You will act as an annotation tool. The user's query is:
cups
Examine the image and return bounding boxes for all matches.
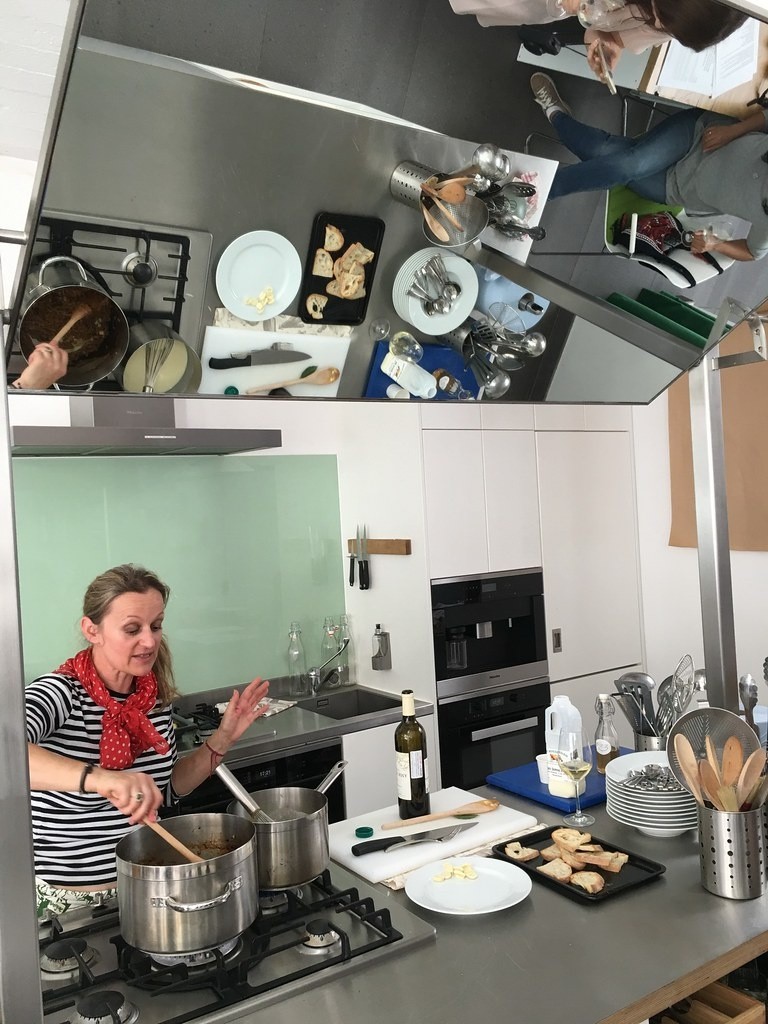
[536,755,550,784]
[386,384,410,399]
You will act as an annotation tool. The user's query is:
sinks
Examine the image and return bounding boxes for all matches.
[289,686,402,720]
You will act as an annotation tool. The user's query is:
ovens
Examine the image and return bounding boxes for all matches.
[158,736,347,826]
[431,567,552,792]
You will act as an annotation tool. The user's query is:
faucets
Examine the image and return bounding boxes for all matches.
[307,666,343,696]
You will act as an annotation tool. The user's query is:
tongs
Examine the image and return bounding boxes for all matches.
[740,657,768,747]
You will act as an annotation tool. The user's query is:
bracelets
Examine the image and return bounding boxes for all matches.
[80,761,97,794]
[204,740,224,778]
[11,382,22,389]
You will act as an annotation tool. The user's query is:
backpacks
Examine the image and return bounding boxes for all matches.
[613,211,724,288]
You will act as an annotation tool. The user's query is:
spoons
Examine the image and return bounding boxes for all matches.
[432,256,461,297]
[420,268,437,315]
[426,262,457,300]
[382,800,498,830]
[409,281,453,315]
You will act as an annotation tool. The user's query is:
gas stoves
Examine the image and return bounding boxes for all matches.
[5,218,191,394]
[171,703,276,754]
[36,861,435,1024]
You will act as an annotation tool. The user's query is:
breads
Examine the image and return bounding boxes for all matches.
[305,225,375,320]
[505,827,629,894]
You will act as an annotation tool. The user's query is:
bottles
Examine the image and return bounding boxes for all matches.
[545,694,620,798]
[394,691,431,820]
[321,615,357,689]
[288,623,308,696]
[372,624,386,658]
[379,352,470,400]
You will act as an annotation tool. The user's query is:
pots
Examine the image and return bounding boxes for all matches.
[14,254,203,396]
[116,788,327,954]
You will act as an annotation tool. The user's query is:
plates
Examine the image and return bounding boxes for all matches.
[392,247,480,337]
[405,856,533,915]
[215,211,387,326]
[605,751,698,837]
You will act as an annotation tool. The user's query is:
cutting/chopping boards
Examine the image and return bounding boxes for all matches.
[198,325,352,398]
[328,786,537,885]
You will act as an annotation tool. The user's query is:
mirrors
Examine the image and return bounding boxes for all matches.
[6,0,768,403]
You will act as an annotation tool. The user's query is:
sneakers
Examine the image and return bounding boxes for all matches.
[530,73,570,123]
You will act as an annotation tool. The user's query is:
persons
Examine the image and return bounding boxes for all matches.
[6,342,69,389]
[449,0,750,82]
[25,562,271,923]
[530,72,768,261]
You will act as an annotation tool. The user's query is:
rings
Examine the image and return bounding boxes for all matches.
[45,347,53,352]
[134,792,144,801]
[709,131,711,134]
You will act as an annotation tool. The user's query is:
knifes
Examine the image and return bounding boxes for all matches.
[209,350,313,370]
[351,822,478,857]
[349,526,370,590]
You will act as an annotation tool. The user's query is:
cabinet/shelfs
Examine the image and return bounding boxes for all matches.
[334,406,647,815]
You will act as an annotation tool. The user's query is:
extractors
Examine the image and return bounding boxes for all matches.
[9,396,284,458]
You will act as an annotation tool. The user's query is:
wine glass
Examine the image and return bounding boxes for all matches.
[681,221,734,247]
[546,0,625,30]
[558,727,596,827]
[369,317,423,364]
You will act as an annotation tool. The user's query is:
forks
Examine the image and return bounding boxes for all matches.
[231,342,292,360]
[385,827,462,852]
